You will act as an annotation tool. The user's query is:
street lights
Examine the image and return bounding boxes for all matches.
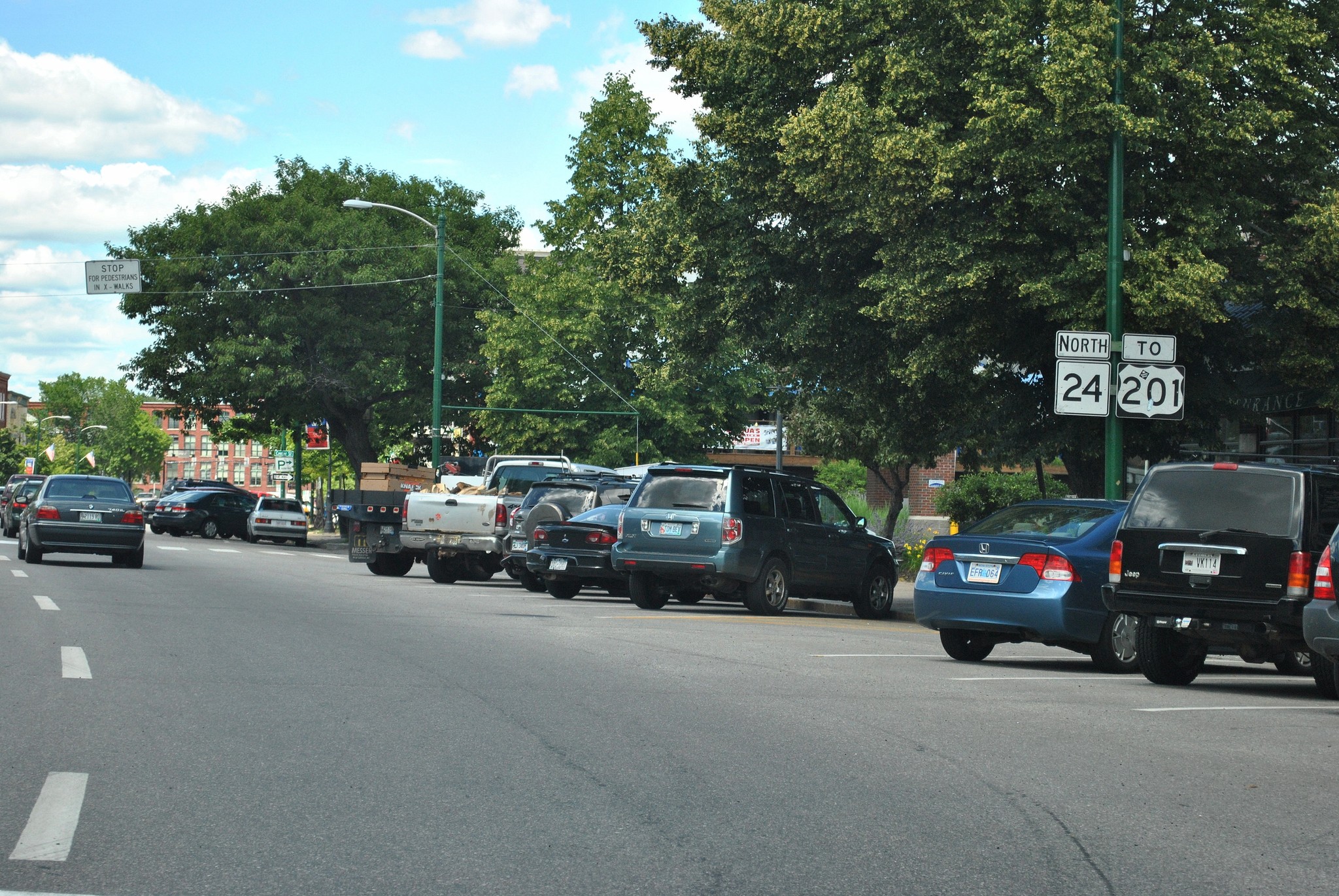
[341,199,449,472]
[34,411,72,473]
[75,424,108,474]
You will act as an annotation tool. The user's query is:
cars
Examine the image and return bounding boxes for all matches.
[134,476,277,541]
[0,474,47,537]
[911,498,1140,673]
[12,474,145,568]
[1300,521,1338,695]
[612,462,902,616]
[246,497,308,546]
[530,502,635,600]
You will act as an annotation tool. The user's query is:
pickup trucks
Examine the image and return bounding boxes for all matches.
[401,453,623,589]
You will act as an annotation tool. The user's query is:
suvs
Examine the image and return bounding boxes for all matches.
[1102,462,1339,687]
[503,478,639,590]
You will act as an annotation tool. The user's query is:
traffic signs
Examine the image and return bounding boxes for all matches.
[273,473,294,482]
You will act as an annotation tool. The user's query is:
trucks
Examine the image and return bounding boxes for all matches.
[327,451,497,586]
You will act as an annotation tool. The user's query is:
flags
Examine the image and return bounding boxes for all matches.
[46,444,55,461]
[86,450,96,468]
